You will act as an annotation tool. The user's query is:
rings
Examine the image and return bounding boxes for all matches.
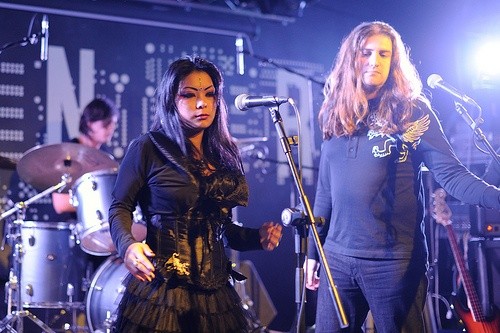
[276,242,279,246]
[134,260,138,265]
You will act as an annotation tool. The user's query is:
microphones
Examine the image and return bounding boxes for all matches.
[427,74,479,106]
[234,94,291,110]
[40,14,49,61]
[235,34,245,76]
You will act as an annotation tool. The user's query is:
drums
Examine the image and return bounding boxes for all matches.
[84,253,131,333]
[7,219,101,311]
[69,168,149,257]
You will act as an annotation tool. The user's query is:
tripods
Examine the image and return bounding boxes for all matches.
[0,178,71,332]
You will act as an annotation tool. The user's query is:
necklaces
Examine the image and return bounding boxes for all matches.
[194,157,208,168]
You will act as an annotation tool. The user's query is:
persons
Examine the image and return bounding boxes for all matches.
[107,56,284,333]
[303,20,500,333]
[52,95,115,216]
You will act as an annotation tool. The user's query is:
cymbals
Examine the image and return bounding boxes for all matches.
[17,142,121,191]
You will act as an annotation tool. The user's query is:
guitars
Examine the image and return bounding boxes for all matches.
[431,188,500,333]
